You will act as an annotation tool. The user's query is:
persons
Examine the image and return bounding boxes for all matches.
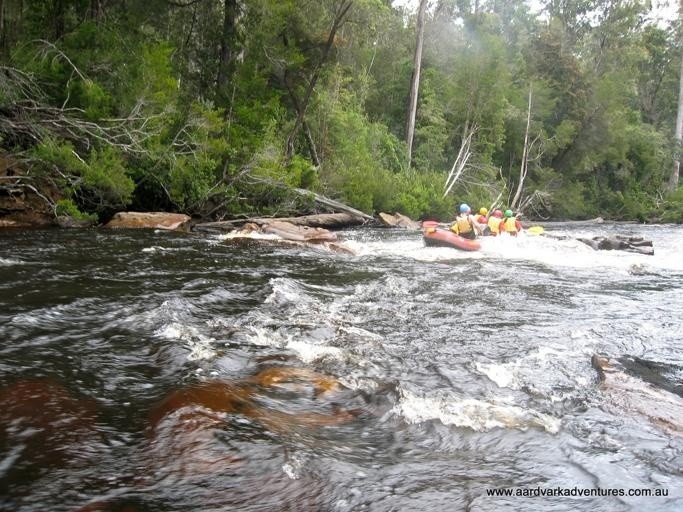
[449,203,522,240]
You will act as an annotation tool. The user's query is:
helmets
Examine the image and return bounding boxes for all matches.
[493,210,503,217]
[480,207,487,215]
[505,208,513,215]
[460,204,470,213]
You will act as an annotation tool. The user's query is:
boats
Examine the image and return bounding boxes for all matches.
[423,226,482,252]
